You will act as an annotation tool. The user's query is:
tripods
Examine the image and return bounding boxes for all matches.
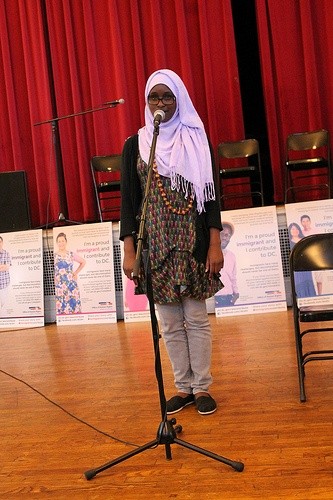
[33,104,117,229]
[83,124,244,481]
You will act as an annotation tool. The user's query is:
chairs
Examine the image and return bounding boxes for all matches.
[89,154,121,222]
[217,138,264,211]
[284,129,333,204]
[289,233,333,402]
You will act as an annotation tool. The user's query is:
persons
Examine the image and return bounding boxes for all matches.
[208,222,239,307]
[54,232,86,316]
[286,215,323,300]
[119,69,226,415]
[0,236,12,310]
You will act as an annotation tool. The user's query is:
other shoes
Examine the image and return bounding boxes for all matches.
[193,393,218,415]
[165,393,194,415]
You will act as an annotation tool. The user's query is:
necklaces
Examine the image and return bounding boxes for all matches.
[152,155,195,214]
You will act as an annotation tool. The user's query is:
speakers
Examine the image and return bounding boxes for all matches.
[0,170,32,234]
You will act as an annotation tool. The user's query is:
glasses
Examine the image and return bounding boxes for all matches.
[147,95,176,105]
[219,231,232,240]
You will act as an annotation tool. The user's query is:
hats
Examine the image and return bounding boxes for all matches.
[220,221,235,236]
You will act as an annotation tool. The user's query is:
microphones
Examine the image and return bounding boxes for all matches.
[103,99,125,105]
[153,110,166,125]
